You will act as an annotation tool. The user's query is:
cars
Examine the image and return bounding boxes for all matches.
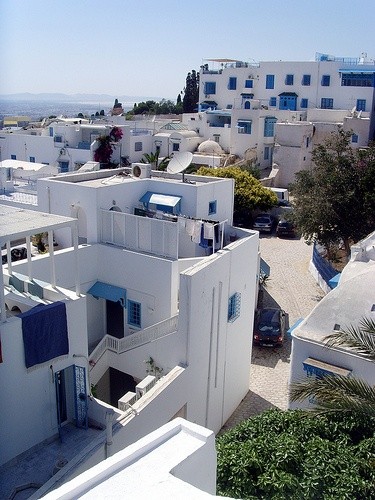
[232,210,251,228]
[276,218,297,238]
[253,213,275,232]
[253,308,285,347]
[278,198,294,209]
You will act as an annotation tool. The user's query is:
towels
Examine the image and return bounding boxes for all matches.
[154,210,222,249]
